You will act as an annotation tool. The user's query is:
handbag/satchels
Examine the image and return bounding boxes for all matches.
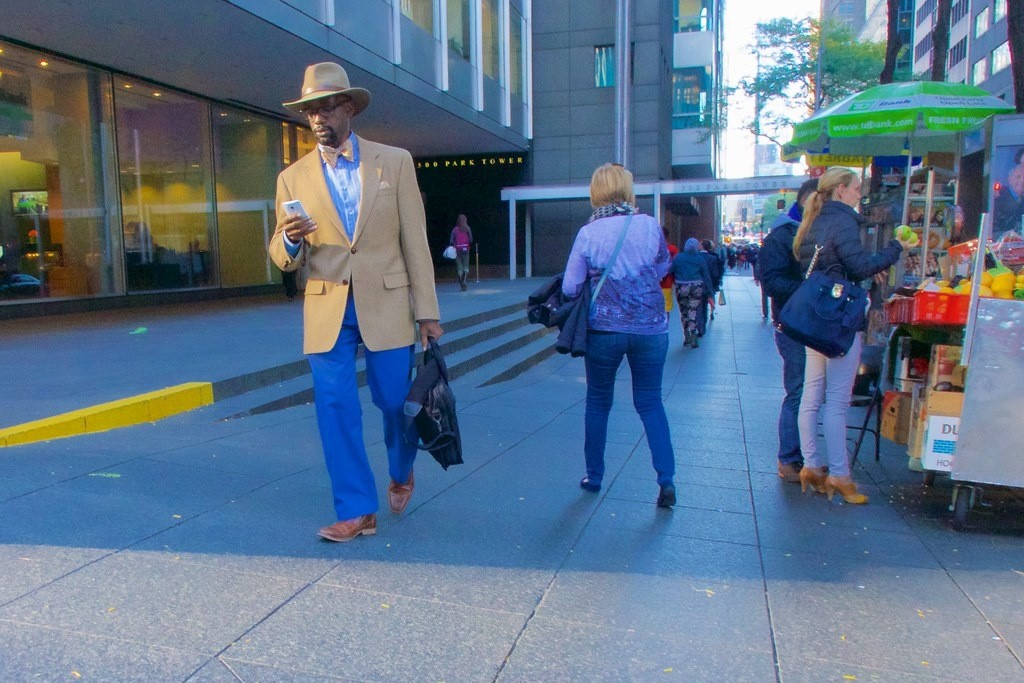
[442,242,457,260]
[775,263,869,359]
[403,337,464,471]
[718,289,726,306]
[705,301,712,332]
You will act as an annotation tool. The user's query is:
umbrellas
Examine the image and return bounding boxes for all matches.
[780,139,871,216]
[791,81,1014,286]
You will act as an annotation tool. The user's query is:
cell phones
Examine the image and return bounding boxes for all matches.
[281,200,309,219]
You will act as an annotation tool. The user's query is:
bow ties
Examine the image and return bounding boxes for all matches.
[321,138,355,168]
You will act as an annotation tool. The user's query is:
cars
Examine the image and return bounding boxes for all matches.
[0,274,50,297]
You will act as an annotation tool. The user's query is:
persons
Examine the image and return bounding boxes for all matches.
[450,214,473,292]
[792,168,920,504]
[661,225,777,348]
[562,164,677,507]
[268,61,443,543]
[757,178,830,485]
[906,209,945,277]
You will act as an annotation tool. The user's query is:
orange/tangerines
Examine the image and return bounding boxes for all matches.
[932,272,1024,300]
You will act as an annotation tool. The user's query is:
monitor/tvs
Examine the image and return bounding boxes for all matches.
[9,188,50,217]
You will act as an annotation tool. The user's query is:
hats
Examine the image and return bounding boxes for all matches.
[282,62,373,122]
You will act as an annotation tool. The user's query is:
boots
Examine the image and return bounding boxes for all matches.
[458,271,467,291]
[690,335,699,347]
[683,331,691,345]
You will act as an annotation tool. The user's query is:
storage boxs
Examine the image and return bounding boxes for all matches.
[948,238,994,262]
[881,336,966,472]
[884,291,971,326]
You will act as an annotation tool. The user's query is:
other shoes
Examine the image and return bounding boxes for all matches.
[777,459,800,482]
[657,481,677,508]
[580,476,601,492]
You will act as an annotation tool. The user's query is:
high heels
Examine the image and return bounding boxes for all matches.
[799,466,837,494]
[824,477,869,504]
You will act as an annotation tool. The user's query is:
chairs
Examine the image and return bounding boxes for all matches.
[815,345,886,471]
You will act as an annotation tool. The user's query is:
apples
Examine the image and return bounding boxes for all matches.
[894,225,918,245]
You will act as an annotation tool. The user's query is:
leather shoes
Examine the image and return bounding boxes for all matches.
[316,513,377,542]
[387,469,413,513]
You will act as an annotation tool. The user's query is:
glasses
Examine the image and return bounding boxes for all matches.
[299,99,352,121]
